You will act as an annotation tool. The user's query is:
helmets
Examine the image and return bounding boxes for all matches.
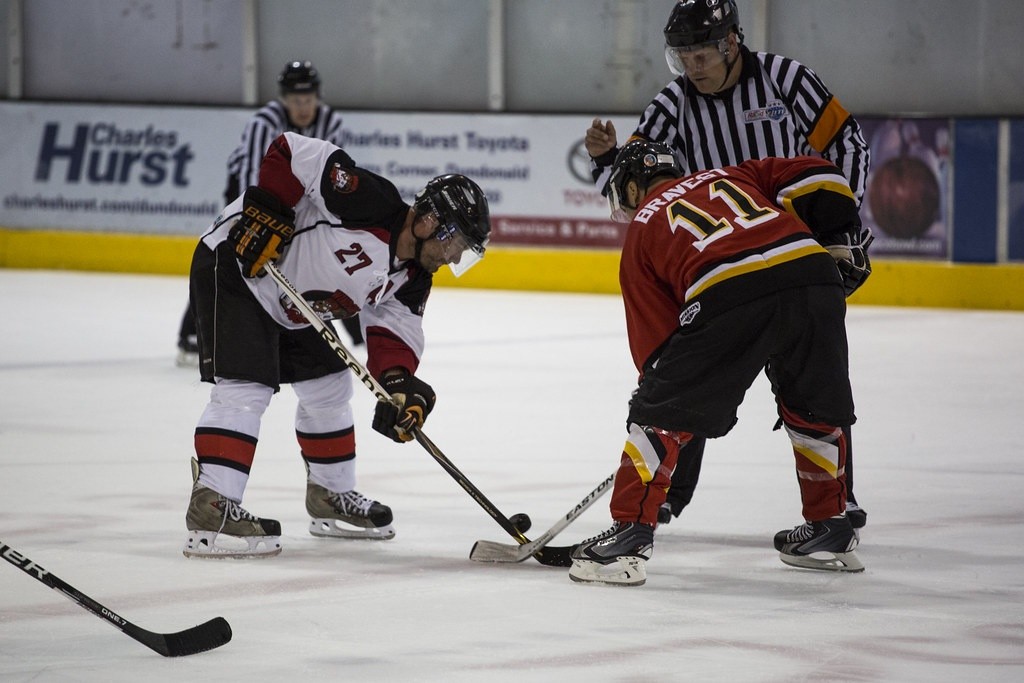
[663,0,739,47]
[601,139,685,203]
[276,61,321,95]
[415,173,491,258]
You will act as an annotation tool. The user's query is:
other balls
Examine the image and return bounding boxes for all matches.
[509,513,532,534]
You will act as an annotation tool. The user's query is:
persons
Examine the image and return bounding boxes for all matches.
[178,59,345,354]
[569,140,871,566]
[186,131,491,537]
[585,0,871,528]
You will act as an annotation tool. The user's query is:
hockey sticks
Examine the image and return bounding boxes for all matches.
[468,248,851,564]
[1,539,235,659]
[239,218,575,568]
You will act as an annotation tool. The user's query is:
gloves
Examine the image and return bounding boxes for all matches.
[227,186,296,279]
[816,226,876,297]
[372,374,436,443]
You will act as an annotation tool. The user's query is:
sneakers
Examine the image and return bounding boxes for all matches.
[774,511,865,574]
[183,456,282,559]
[301,450,396,540]
[569,520,655,587]
